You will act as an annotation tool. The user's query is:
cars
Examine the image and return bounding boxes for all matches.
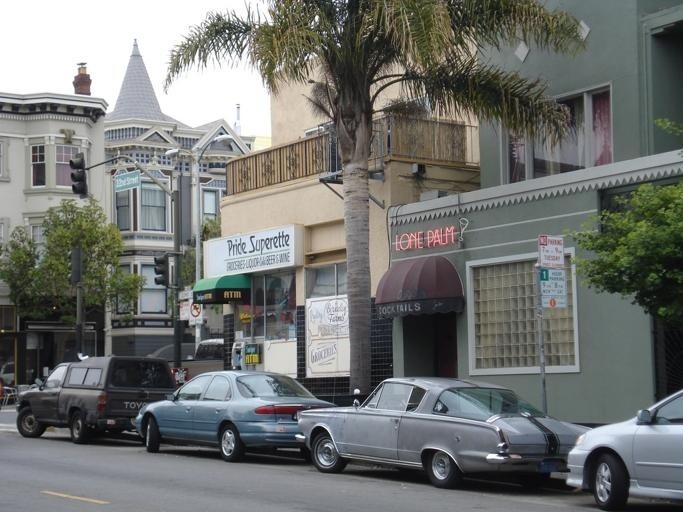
[564,388,682,511]
[1,361,15,386]
[134,370,340,462]
[295,374,593,486]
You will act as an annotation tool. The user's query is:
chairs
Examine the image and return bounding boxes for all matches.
[2,386,18,406]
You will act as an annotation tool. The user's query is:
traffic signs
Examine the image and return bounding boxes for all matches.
[114,169,141,192]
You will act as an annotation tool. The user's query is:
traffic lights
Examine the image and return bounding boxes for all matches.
[153,254,169,287]
[68,247,82,283]
[70,154,88,196]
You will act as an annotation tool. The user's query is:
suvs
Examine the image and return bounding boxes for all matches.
[15,355,180,446]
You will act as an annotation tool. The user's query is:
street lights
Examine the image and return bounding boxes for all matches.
[164,133,235,360]
